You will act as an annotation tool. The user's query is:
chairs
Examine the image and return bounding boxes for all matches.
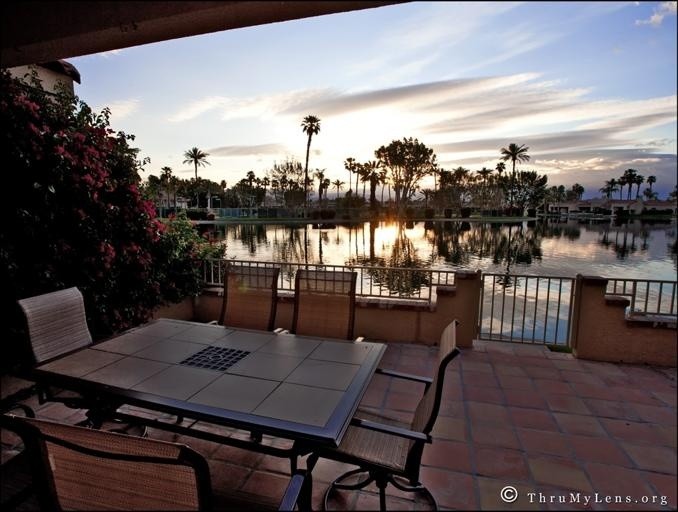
[0,259,461,511]
